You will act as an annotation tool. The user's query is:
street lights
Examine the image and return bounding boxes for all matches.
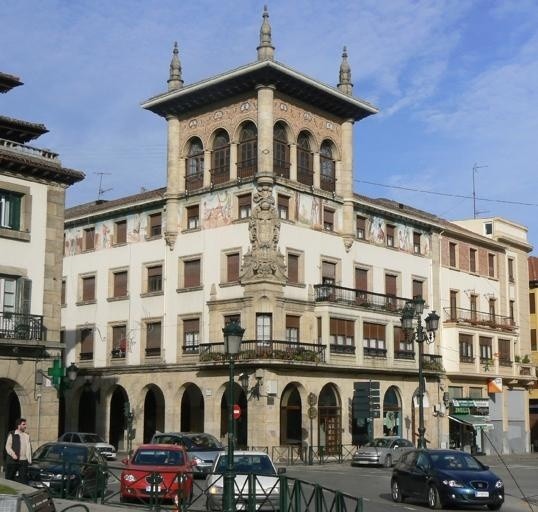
[221,319,245,511]
[401,294,441,452]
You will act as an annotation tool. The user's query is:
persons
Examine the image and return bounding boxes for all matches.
[5,418,32,484]
[448,457,463,468]
[376,441,384,447]
[237,458,252,472]
[164,453,181,464]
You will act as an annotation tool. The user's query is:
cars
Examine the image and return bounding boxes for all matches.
[207,451,288,511]
[148,430,224,478]
[17,442,108,501]
[352,436,414,469]
[120,444,197,505]
[59,431,116,460]
[392,449,506,512]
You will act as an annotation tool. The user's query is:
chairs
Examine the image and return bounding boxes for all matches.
[16,487,89,512]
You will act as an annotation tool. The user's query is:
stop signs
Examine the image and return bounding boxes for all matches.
[233,405,241,419]
